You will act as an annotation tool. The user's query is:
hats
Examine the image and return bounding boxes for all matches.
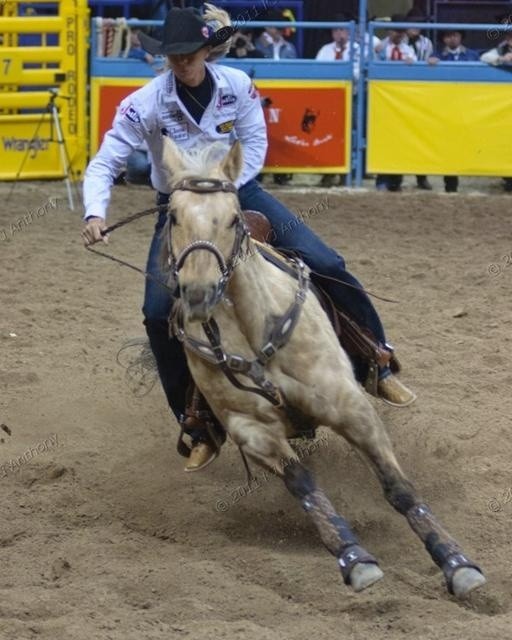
[254,8,291,22]
[401,7,430,22]
[137,7,234,57]
[386,12,407,32]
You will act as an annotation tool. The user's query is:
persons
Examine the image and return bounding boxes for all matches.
[80,7,418,472]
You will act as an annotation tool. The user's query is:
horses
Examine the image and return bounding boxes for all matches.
[158,133,489,600]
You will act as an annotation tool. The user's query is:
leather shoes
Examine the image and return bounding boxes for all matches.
[183,439,216,473]
[417,178,430,190]
[362,374,416,408]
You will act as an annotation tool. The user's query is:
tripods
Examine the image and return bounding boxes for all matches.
[6,104,81,210]
